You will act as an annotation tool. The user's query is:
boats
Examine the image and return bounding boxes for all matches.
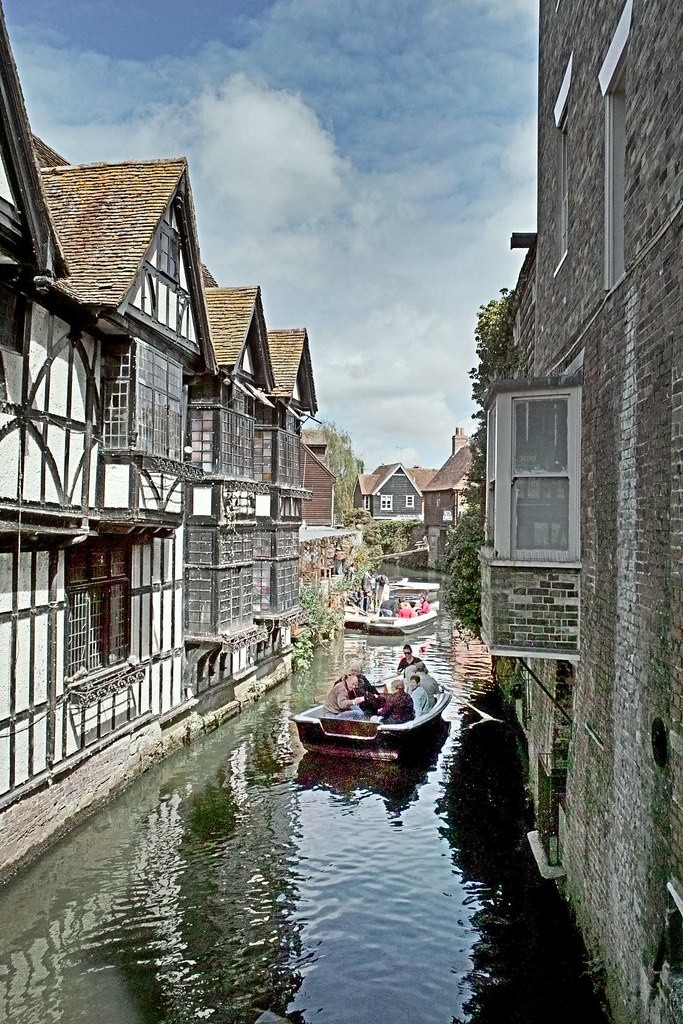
[343,608,439,636]
[290,675,452,761]
[390,581,440,594]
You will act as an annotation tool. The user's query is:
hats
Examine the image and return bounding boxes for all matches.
[416,662,426,669]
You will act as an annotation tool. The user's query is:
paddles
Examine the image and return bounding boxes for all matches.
[436,683,494,720]
[313,674,399,701]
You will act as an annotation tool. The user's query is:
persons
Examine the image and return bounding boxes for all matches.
[362,569,430,619]
[324,643,439,725]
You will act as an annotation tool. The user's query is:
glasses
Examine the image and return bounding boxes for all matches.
[404,652,411,654]
